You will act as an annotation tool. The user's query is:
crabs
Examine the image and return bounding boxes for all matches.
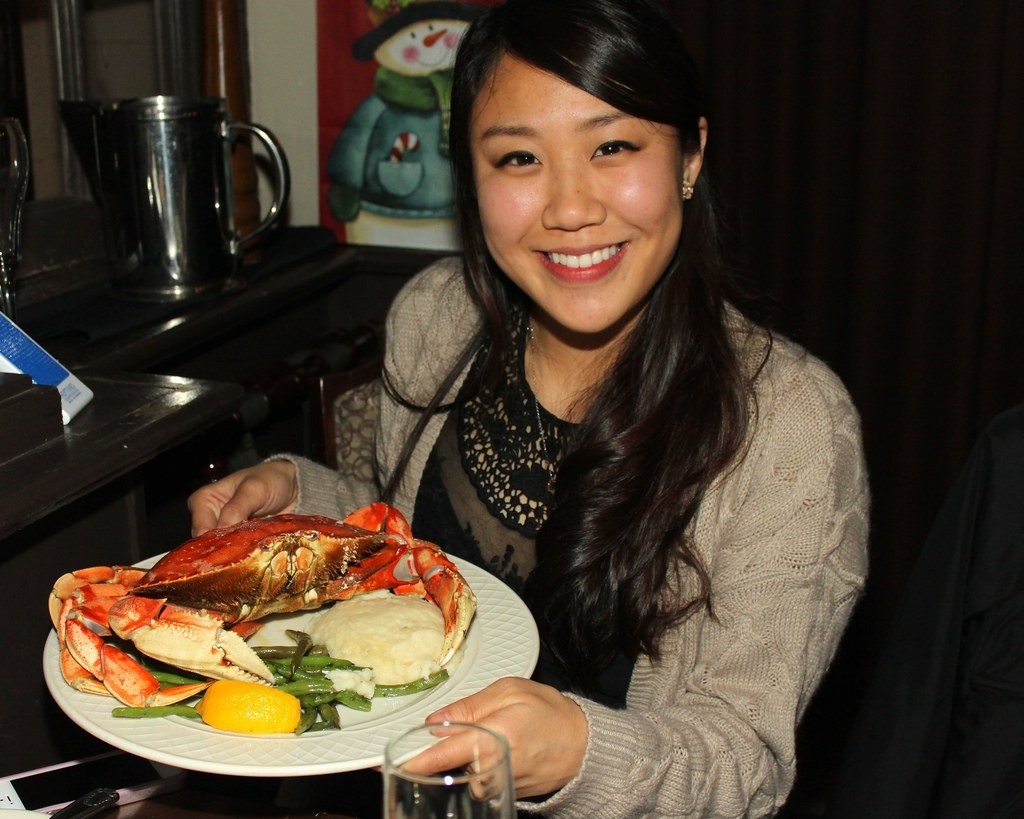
[48,502,477,707]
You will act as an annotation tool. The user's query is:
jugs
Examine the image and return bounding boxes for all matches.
[56,91,289,306]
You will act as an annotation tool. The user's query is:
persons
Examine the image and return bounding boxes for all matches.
[188,0,869,819]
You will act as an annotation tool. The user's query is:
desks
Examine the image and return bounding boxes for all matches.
[2,246,353,817]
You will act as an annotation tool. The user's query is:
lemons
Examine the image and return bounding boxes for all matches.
[194,679,301,736]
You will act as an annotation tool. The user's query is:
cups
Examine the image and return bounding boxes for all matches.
[382,720,518,819]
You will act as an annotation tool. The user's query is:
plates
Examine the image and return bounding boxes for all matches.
[41,550,540,779]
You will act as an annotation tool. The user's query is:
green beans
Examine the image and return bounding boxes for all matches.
[110,628,450,737]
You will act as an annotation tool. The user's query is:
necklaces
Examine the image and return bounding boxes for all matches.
[526,316,559,495]
